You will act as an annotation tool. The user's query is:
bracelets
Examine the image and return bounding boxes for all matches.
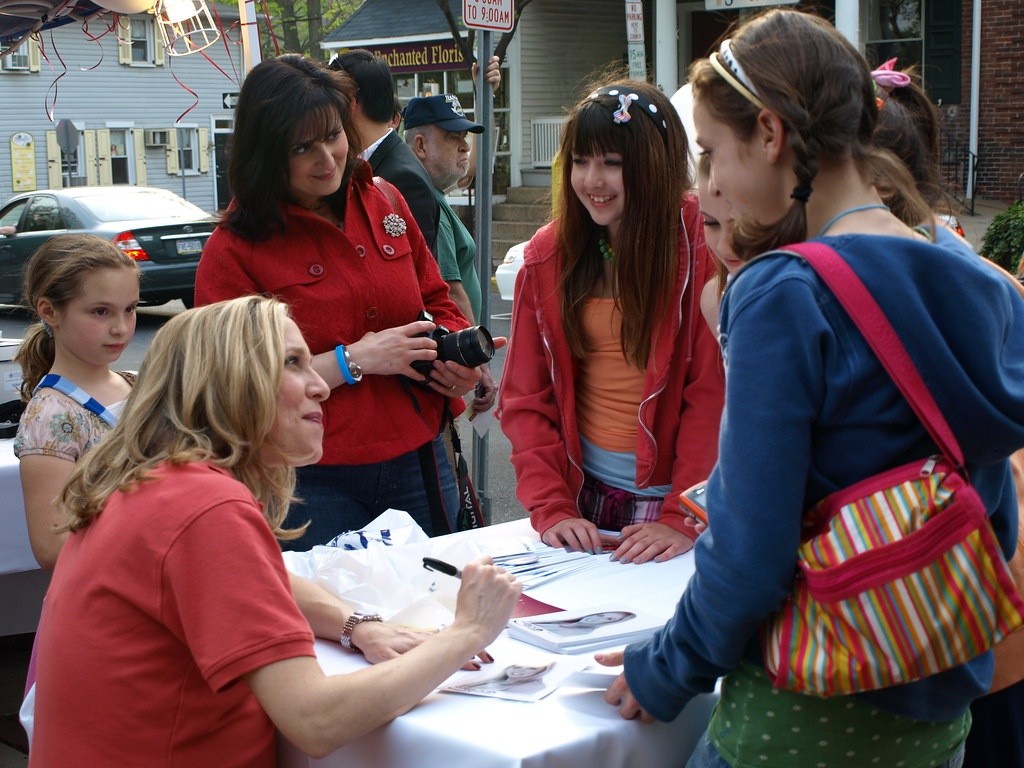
[335,345,358,385]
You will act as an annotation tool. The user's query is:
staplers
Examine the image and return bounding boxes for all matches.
[600,533,619,551]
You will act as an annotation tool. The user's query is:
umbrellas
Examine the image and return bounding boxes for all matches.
[670,82,703,194]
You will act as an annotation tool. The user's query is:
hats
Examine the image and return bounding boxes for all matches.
[403,94,485,134]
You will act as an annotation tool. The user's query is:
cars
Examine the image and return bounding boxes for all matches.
[0,185,221,309]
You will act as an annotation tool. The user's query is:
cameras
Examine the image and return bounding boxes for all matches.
[398,310,495,393]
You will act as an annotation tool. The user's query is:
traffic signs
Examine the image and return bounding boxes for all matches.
[222,92,241,110]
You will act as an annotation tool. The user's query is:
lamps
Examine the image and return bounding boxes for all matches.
[152,0,221,57]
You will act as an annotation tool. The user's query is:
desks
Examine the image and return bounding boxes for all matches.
[19,518,722,768]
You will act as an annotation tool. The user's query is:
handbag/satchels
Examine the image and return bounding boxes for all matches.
[761,241,1024,700]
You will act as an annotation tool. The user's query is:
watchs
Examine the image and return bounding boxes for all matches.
[343,349,362,382]
[341,609,384,651]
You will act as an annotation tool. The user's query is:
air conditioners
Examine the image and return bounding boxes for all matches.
[144,129,170,147]
[2,53,30,70]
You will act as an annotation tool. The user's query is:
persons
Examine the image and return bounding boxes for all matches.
[493,81,723,565]
[462,662,556,695]
[25,297,523,768]
[194,50,507,552]
[594,12,1024,768]
[11,234,140,567]
[530,611,637,638]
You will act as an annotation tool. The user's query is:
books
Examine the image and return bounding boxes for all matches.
[437,597,667,703]
[492,536,601,592]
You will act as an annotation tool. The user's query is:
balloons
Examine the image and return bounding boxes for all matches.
[90,0,156,14]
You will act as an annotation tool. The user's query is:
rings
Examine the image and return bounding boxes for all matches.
[450,385,456,391]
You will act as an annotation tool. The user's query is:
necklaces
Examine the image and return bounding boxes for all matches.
[815,205,890,239]
[301,201,326,211]
[599,238,617,265]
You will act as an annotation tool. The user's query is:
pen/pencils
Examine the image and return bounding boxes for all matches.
[423,558,461,578]
